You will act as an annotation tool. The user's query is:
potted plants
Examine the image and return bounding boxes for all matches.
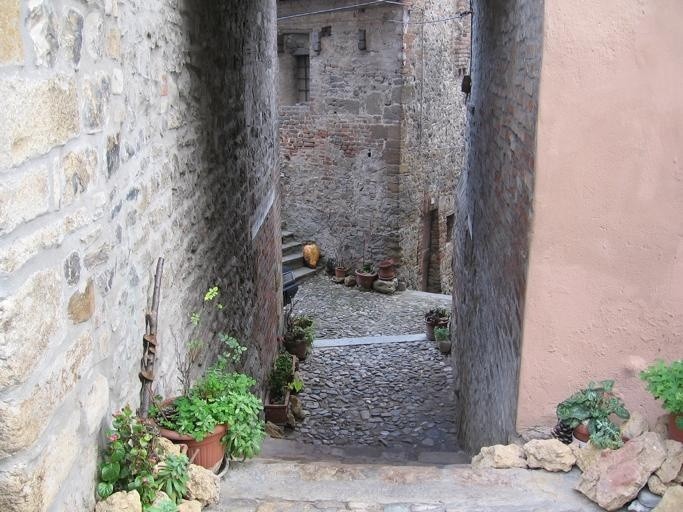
[637,354,682,446]
[258,348,300,431]
[129,271,269,487]
[279,290,315,361]
[327,257,396,293]
[422,305,451,342]
[553,378,631,453]
[430,323,452,353]
